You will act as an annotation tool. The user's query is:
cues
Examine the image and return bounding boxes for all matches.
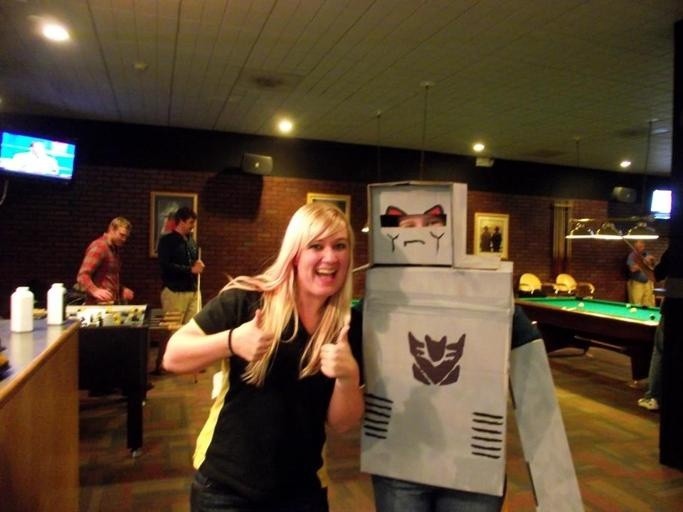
[192,247,202,383]
[574,335,626,352]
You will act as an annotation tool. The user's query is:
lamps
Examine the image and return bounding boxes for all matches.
[558,117,663,244]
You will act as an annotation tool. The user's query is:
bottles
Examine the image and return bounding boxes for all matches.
[10,287,32,332]
[46,283,67,325]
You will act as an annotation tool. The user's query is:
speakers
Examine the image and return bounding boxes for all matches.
[240,153,273,176]
[612,186,637,203]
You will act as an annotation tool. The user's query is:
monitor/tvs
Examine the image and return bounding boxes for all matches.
[0,127,78,186]
[646,184,672,223]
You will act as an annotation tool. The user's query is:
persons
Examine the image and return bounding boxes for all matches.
[161,200,365,508]
[347,215,541,511]
[491,225,502,252]
[480,224,491,252]
[156,206,207,374]
[637,233,673,410]
[14,142,59,176]
[76,216,156,392]
[626,240,655,308]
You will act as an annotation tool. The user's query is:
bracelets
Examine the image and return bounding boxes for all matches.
[229,328,234,357]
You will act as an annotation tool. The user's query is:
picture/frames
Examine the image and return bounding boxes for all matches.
[149,189,199,261]
[304,191,352,225]
[472,210,509,260]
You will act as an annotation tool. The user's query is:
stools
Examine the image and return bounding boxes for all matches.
[651,287,668,307]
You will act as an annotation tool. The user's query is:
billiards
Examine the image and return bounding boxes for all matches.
[649,313,655,320]
[631,307,637,312]
[626,304,632,308]
[578,302,584,308]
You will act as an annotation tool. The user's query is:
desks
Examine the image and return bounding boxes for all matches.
[0,316,86,512]
[509,293,665,387]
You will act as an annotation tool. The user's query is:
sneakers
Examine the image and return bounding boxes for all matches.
[638,398,660,410]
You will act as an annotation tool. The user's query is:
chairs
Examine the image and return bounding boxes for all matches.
[554,270,597,301]
[516,272,561,299]
